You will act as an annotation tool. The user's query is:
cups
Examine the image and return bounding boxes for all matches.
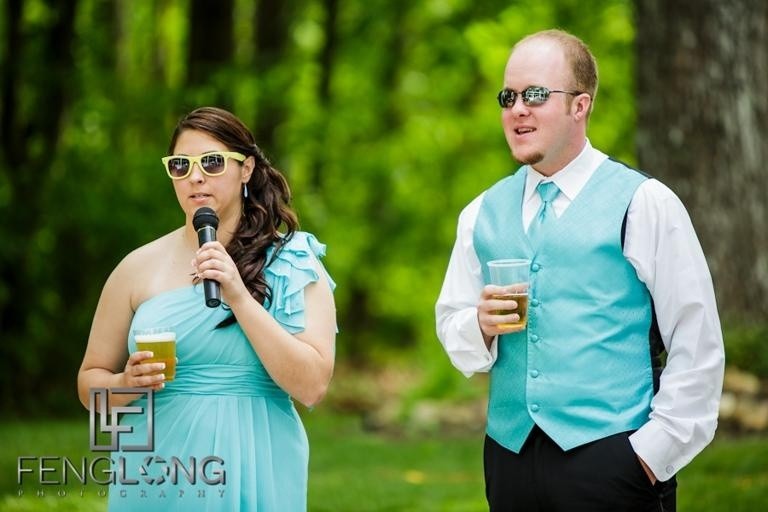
[486,258,532,329]
[131,326,177,383]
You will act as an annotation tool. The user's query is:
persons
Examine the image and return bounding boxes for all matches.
[76,105,340,512]
[435,30,727,512]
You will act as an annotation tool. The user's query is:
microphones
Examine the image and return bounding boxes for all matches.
[192,207,221,308]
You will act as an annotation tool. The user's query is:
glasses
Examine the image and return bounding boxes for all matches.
[495,85,593,110]
[161,149,248,181]
